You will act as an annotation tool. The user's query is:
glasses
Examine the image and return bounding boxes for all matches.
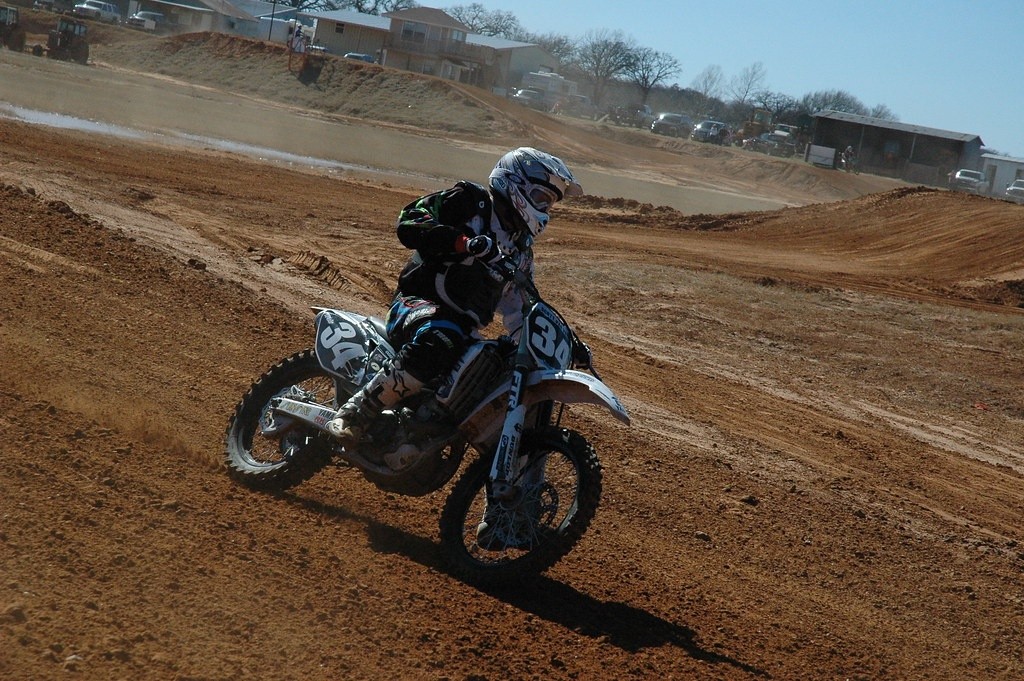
[526,184,558,214]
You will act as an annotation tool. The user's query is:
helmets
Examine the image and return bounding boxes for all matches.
[488,147,583,235]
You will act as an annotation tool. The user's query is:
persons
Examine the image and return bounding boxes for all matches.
[707,122,737,145]
[294,23,305,37]
[844,144,856,169]
[329,147,583,548]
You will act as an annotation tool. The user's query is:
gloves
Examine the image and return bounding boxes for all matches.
[465,235,502,269]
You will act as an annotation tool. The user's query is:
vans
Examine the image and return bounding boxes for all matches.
[774,124,795,138]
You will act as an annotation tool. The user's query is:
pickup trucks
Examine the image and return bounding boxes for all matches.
[613,103,657,128]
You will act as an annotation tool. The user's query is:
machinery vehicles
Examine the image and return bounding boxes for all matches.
[45,14,89,64]
[0,1,26,53]
[735,108,778,148]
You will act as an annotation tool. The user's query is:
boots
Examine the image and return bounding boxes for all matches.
[328,358,427,450]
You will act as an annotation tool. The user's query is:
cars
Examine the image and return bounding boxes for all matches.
[691,121,725,142]
[748,132,795,158]
[344,51,377,63]
[1005,179,1024,204]
[513,89,544,110]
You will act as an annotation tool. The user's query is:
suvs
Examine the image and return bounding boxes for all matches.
[951,169,990,195]
[128,11,165,32]
[72,0,121,25]
[650,115,693,138]
[562,94,600,121]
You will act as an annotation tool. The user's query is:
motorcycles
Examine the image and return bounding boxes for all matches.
[222,238,633,585]
[839,150,860,177]
